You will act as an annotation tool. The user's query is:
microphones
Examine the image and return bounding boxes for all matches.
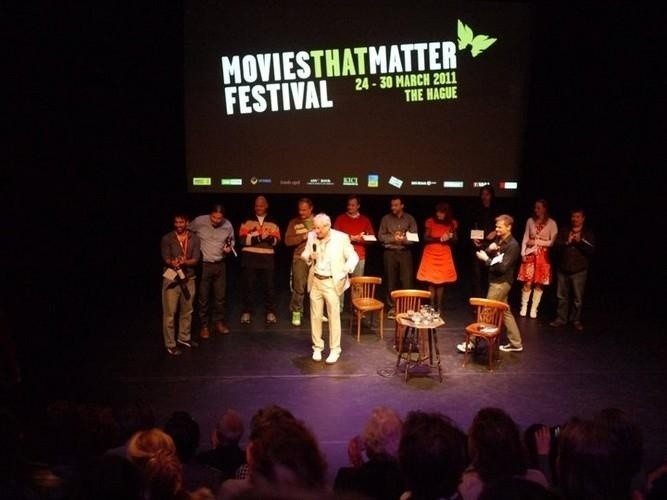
[313,244,316,265]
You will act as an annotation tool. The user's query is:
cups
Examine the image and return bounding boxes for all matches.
[406,305,441,326]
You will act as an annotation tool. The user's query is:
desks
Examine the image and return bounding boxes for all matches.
[396,313,445,383]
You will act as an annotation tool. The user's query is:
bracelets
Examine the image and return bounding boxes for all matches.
[188,260,193,266]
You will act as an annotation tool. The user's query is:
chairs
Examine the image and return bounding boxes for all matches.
[349,276,384,342]
[391,290,429,350]
[466,297,508,372]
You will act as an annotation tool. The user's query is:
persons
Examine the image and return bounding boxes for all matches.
[414,203,458,313]
[299,213,360,365]
[331,195,376,316]
[0,396,667,499]
[516,199,558,319]
[547,206,595,330]
[237,195,281,329]
[284,197,328,326]
[187,203,235,339]
[455,214,523,353]
[161,214,200,357]
[377,196,419,320]
[460,186,497,314]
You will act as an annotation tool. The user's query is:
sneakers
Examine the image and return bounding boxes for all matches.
[456,319,584,353]
[387,309,395,319]
[165,311,342,365]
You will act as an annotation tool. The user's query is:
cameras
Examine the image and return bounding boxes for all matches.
[551,425,561,438]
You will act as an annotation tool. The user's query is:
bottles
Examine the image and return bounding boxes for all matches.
[172,257,187,282]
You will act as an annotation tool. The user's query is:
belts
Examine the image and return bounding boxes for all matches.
[314,273,332,280]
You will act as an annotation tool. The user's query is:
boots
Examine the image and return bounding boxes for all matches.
[520,289,532,317]
[530,289,544,319]
